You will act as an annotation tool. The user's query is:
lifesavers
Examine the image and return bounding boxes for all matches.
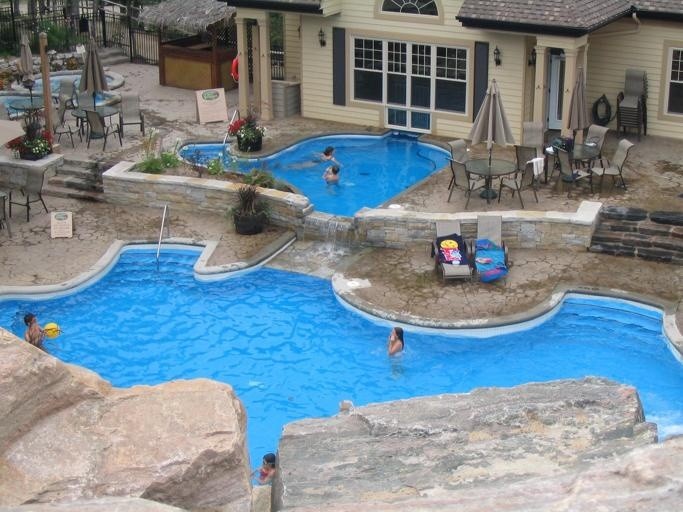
[441,240,458,248]
[232,58,239,80]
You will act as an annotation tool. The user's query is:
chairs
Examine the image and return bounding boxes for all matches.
[0,195,11,238]
[444,67,647,211]
[9,77,144,154]
[9,167,49,222]
[472,215,509,287]
[430,220,472,284]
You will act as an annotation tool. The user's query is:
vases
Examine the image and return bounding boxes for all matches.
[236,134,262,152]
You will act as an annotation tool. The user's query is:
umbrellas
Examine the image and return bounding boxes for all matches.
[78,39,109,111]
[566,63,590,150]
[21,35,36,102]
[467,78,516,166]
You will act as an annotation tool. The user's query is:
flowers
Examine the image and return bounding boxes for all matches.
[226,112,264,144]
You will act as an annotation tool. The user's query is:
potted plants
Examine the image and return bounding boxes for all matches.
[227,185,271,234]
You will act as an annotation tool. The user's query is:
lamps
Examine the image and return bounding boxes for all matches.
[316,26,327,48]
[493,44,501,67]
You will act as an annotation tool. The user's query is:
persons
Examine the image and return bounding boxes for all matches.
[289,146,344,170]
[323,166,340,185]
[24,313,47,352]
[250,453,276,485]
[388,327,404,355]
